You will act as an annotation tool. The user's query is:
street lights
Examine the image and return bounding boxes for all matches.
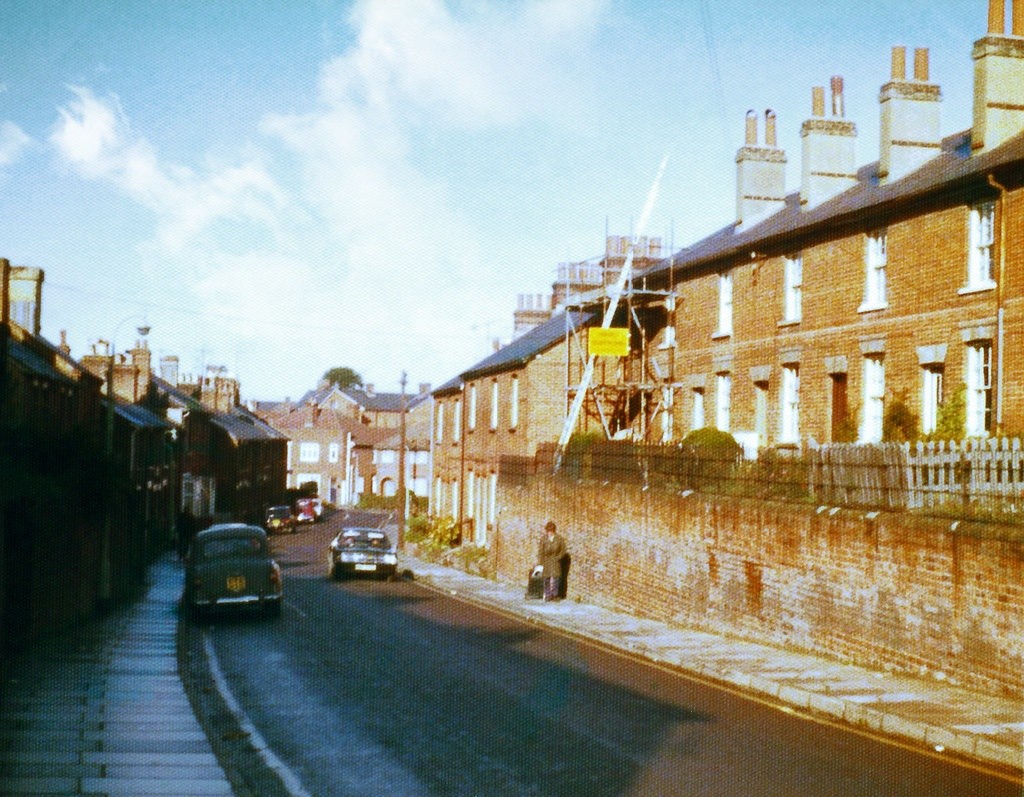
[343,431,355,519]
[101,315,150,599]
[396,445,410,520]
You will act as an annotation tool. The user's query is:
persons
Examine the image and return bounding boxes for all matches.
[174,506,197,562]
[538,522,567,601]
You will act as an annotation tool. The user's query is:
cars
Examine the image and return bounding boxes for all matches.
[184,523,284,618]
[265,506,297,534]
[328,527,399,580]
[298,498,323,522]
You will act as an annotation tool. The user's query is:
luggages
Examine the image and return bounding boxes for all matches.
[525,570,545,600]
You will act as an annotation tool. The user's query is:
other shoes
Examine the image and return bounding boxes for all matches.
[551,596,559,602]
[544,595,552,602]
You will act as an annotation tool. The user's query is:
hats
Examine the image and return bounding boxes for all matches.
[545,521,555,532]
